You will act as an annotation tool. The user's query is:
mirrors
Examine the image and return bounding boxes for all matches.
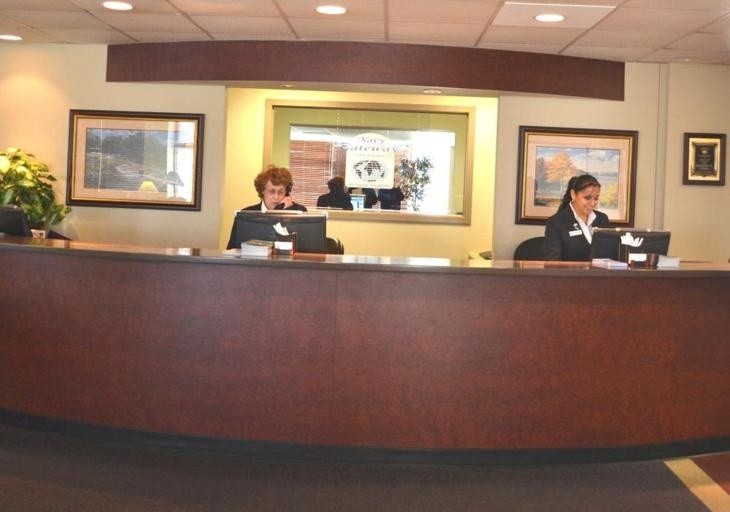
[261,98,476,224]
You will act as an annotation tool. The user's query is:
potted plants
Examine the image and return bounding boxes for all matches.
[0,147,70,238]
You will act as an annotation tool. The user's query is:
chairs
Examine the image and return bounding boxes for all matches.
[514,236,545,260]
[326,236,344,254]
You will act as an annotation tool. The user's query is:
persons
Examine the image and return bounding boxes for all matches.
[227,168,308,250]
[379,188,404,210]
[545,175,610,261]
[316,177,353,210]
[347,187,378,208]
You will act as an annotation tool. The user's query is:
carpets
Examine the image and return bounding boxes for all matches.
[664,452,730,512]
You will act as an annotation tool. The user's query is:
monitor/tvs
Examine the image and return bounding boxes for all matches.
[234,211,329,253]
[589,228,671,262]
[349,194,365,209]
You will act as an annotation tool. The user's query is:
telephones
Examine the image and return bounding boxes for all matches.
[274,183,293,210]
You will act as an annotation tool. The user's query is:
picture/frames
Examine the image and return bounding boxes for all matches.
[684,132,728,185]
[514,125,639,226]
[65,108,204,211]
